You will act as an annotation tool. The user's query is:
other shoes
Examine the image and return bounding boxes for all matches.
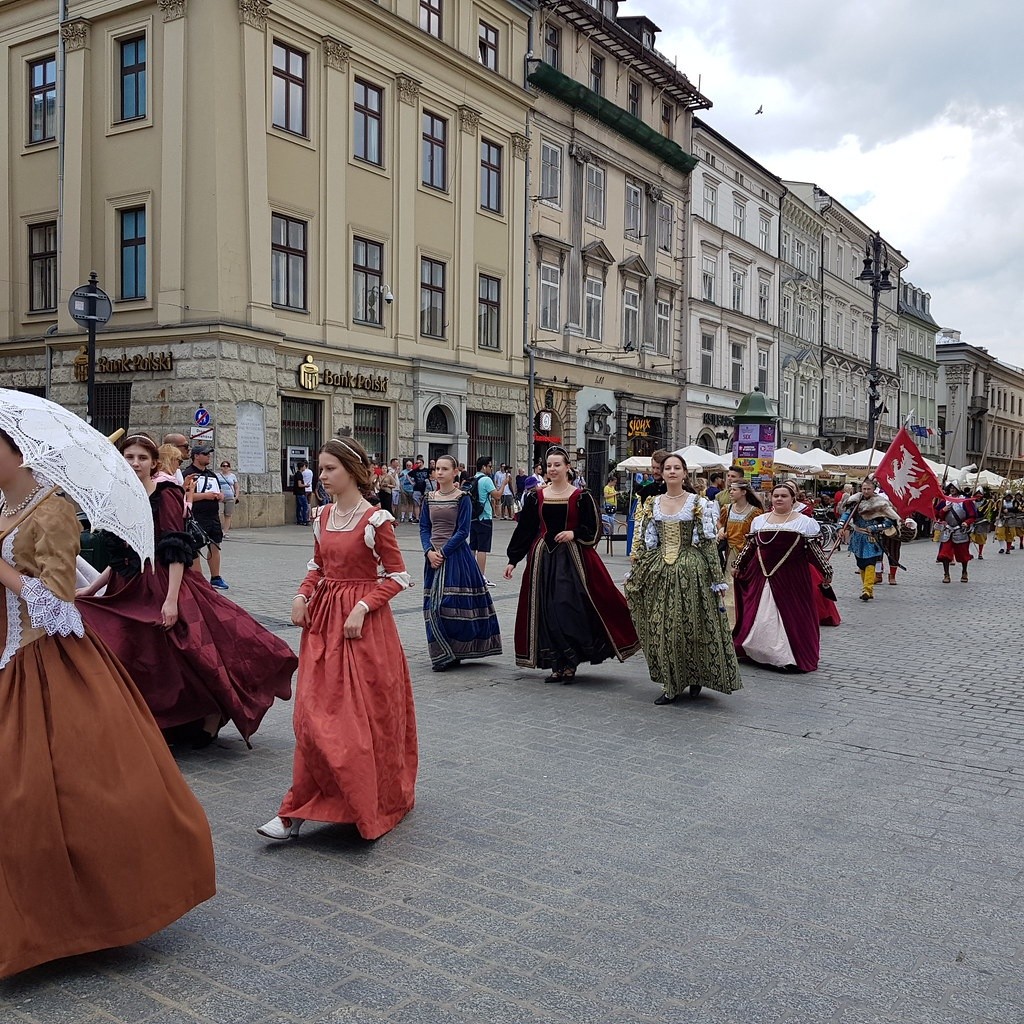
[545,667,562,682]
[654,691,677,705]
[297,521,311,526]
[400,519,404,523]
[689,681,700,697]
[508,517,514,521]
[500,517,509,520]
[192,717,222,750]
[412,519,419,524]
[562,663,576,682]
[483,578,496,587]
[859,542,1024,601]
[409,517,414,522]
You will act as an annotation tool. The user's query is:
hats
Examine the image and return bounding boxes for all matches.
[504,466,513,472]
[191,445,214,458]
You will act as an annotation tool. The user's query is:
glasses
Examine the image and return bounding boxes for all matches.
[487,465,492,469]
[200,452,208,456]
[222,465,230,468]
[176,443,189,450]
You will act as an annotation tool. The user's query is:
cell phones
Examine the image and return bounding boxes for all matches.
[193,475,198,483]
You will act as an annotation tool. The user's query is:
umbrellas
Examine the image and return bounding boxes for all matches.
[616,444,1024,487]
[0,388,155,575]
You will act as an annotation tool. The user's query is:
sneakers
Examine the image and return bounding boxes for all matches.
[210,578,228,589]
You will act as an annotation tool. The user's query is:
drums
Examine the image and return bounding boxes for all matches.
[884,523,917,543]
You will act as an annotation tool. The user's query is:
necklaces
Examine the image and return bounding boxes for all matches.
[734,502,749,514]
[550,484,570,494]
[759,509,794,544]
[438,487,456,495]
[330,497,363,530]
[2,484,44,516]
[665,490,685,499]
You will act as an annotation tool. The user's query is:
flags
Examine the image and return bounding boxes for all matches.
[877,430,945,522]
[910,425,933,438]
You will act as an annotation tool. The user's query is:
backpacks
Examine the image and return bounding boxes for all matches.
[461,476,488,519]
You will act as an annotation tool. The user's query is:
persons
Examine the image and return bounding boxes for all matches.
[294,461,331,526]
[0,429,216,978]
[623,454,745,704]
[371,450,772,631]
[153,433,239,589]
[785,478,1024,601]
[730,484,834,673]
[503,451,642,683]
[258,437,419,840]
[78,434,300,758]
[420,455,503,671]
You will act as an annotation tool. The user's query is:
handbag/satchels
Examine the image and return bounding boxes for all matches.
[186,507,210,549]
[606,506,616,514]
[234,495,240,504]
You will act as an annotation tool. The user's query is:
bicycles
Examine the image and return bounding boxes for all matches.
[817,520,841,552]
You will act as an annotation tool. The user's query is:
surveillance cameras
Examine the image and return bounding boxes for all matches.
[385,293,393,304]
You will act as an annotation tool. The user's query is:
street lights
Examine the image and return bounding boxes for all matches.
[855,230,897,449]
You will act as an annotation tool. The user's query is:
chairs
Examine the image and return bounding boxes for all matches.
[595,514,628,556]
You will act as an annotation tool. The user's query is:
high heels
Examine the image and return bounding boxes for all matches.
[338,820,358,847]
[257,817,305,840]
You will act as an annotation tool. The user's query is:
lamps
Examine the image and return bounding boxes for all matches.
[611,435,617,445]
